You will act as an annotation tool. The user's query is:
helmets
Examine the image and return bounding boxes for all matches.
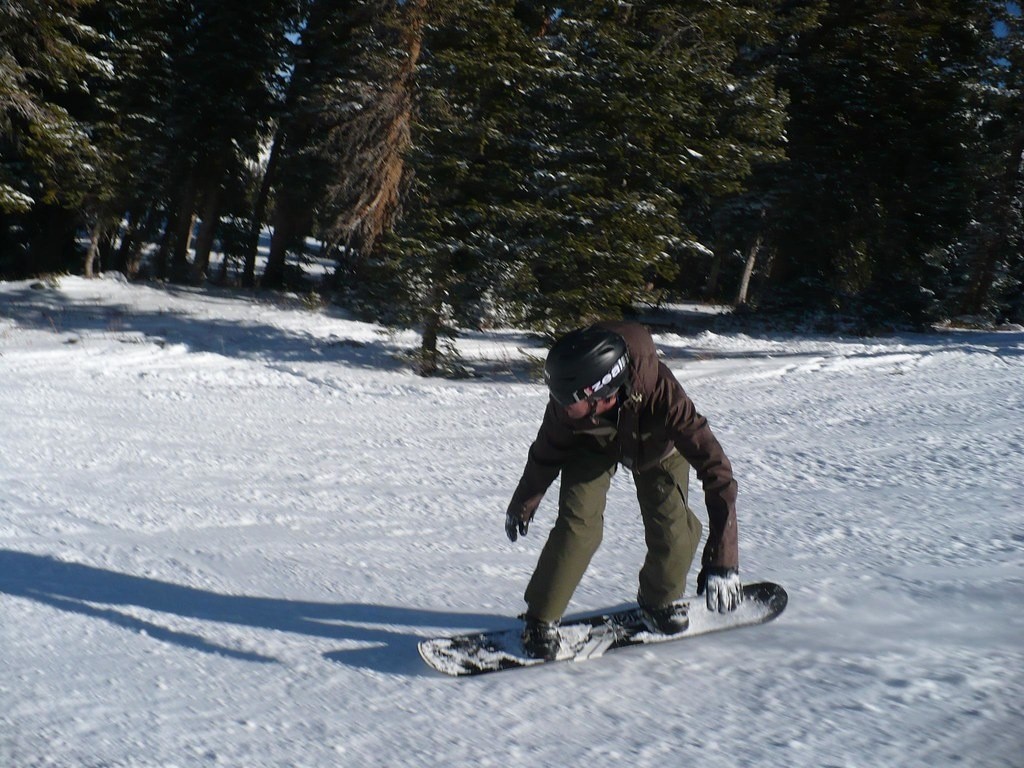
[544,326,633,405]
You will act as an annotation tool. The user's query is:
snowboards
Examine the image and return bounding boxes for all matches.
[415,580,791,680]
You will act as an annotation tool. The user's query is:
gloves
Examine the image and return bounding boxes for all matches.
[697,569,744,613]
[504,511,532,541]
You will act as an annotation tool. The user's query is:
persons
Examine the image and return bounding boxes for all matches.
[506,318,744,660]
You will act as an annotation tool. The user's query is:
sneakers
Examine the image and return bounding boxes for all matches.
[522,612,563,658]
[636,589,689,634]
